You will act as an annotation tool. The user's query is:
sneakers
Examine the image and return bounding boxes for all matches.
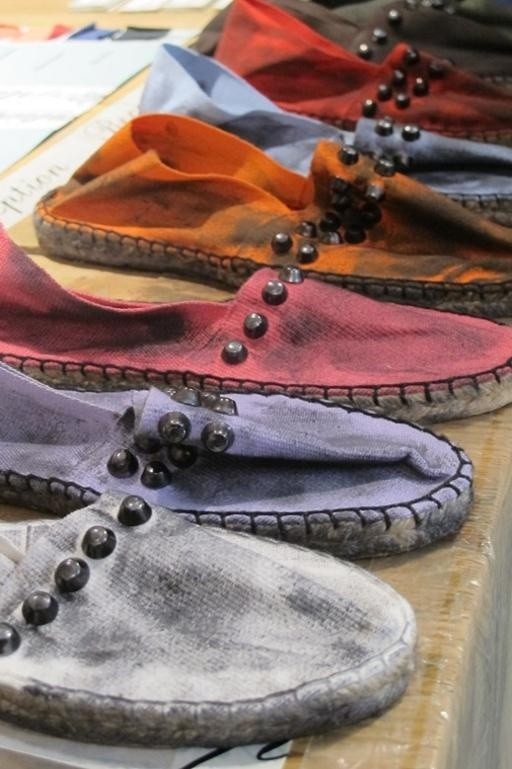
[0,220,512,422]
[213,1,512,143]
[194,0,512,83]
[1,487,419,749]
[31,114,512,319]
[138,42,512,196]
[0,361,474,560]
